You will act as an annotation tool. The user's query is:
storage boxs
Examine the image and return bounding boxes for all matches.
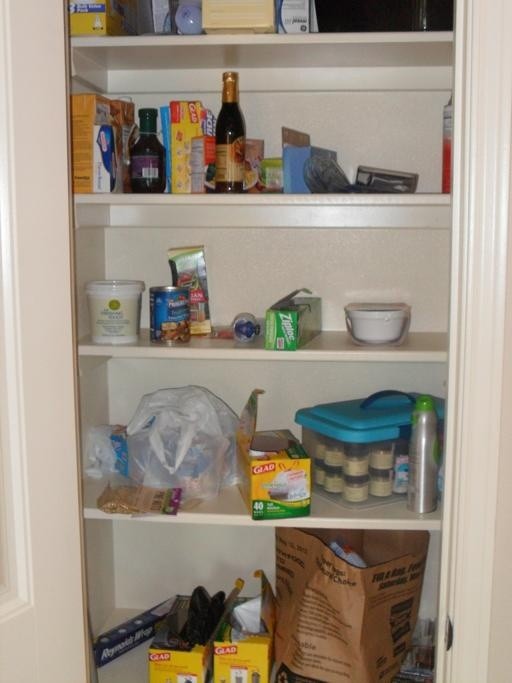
[295,391,445,512]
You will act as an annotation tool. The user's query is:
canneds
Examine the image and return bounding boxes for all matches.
[148,285,192,346]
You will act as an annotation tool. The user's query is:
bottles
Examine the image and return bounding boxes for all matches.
[215,71,246,194]
[311,394,448,514]
[130,107,165,193]
[443,97,452,194]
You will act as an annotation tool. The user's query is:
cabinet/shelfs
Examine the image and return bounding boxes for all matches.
[0,0,512,683]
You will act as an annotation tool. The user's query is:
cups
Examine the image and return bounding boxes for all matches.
[84,278,145,345]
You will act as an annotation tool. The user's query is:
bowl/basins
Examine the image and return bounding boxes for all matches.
[344,307,410,347]
